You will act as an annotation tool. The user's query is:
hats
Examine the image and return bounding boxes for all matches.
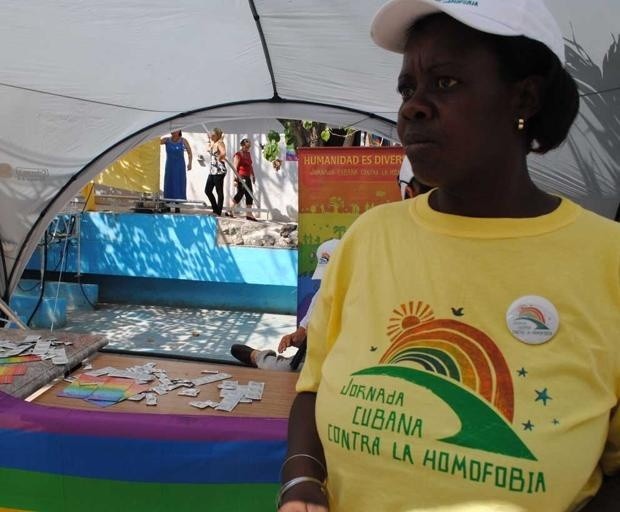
[312,238,340,280]
[369,2,566,65]
[399,155,414,200]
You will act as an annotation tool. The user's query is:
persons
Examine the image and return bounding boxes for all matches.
[230,239,342,371]
[274,0,617,512]
[205,127,225,215]
[398,153,431,200]
[160,130,193,212]
[227,139,259,221]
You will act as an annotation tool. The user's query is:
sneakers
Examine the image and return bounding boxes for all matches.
[230,343,254,364]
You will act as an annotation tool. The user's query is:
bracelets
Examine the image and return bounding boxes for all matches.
[277,480,327,506]
[279,453,326,477]
